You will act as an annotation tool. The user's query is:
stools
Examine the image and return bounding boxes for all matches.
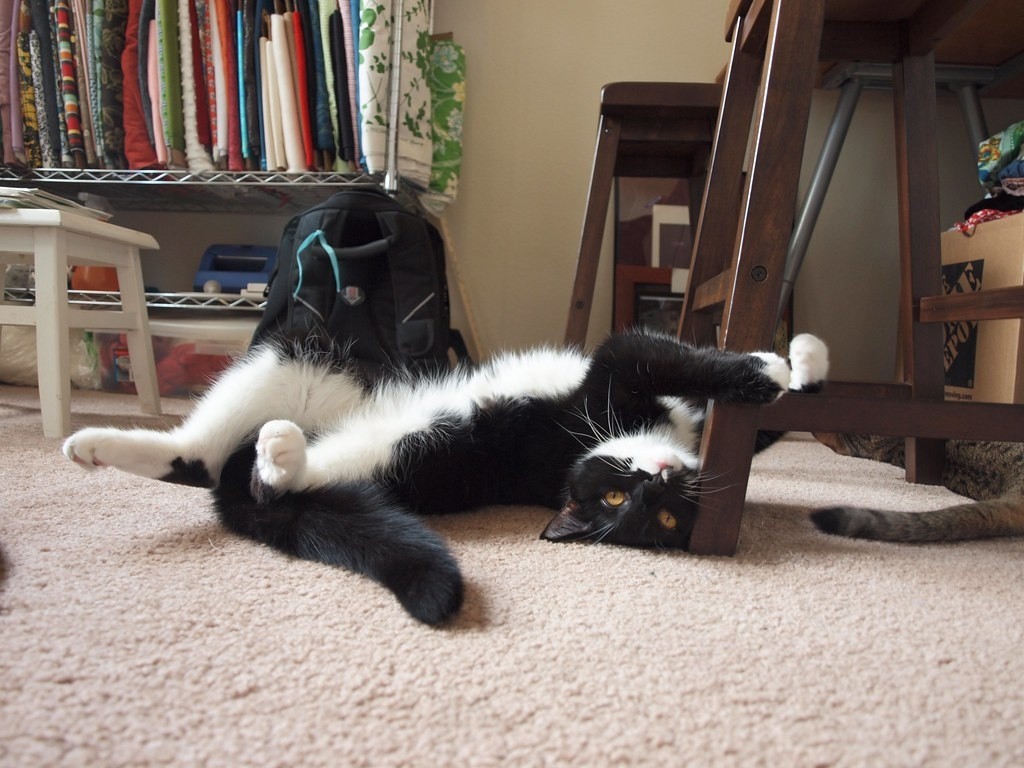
[677,0,1024,558]
[562,81,724,351]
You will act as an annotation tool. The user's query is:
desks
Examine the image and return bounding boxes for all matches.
[713,0,1024,332]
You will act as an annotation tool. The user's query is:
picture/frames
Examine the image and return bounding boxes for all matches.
[611,171,794,365]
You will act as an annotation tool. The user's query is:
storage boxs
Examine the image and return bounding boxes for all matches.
[83,313,262,400]
[893,211,1024,404]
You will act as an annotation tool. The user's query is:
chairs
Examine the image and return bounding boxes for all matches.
[0,207,162,440]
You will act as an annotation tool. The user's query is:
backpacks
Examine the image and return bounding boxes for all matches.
[246,189,468,400]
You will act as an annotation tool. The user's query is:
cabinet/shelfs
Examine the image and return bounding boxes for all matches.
[0,0,435,314]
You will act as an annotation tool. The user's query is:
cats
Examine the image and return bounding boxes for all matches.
[61,327,829,627]
